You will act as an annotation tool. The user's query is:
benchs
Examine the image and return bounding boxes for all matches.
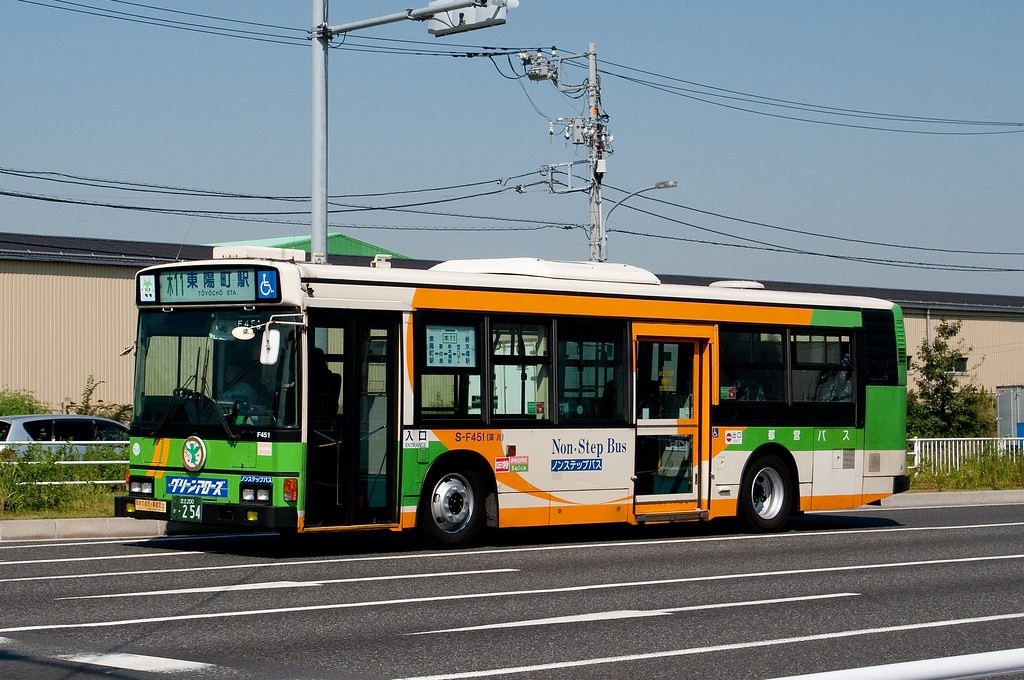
[602,380,663,419]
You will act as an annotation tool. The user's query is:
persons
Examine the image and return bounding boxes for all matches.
[219,362,258,403]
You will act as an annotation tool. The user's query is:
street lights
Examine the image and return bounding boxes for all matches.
[589,176,676,262]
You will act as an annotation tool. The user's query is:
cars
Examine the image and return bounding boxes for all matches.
[0,413,132,460]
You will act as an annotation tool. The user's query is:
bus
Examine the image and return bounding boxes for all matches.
[111,242,916,551]
